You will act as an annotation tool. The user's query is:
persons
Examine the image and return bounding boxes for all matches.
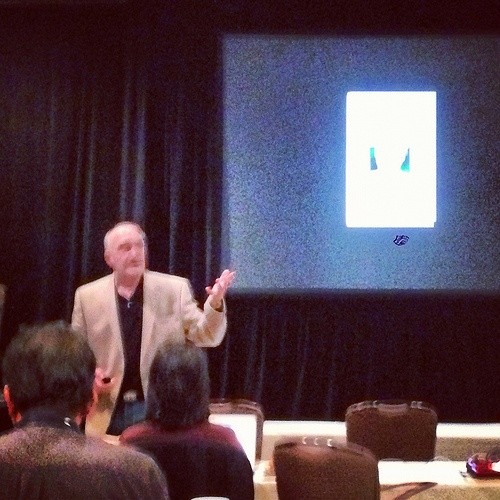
[118,343,255,500]
[0,319,172,500]
[68,221,236,442]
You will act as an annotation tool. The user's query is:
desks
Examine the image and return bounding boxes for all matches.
[261,420,500,461]
[253,459,500,500]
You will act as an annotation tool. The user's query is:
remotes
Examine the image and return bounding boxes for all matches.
[102,377,110,384]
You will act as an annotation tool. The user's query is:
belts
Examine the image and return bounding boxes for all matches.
[117,389,144,403]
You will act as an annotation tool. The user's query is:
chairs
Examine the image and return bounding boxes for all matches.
[344,400,438,460]
[273,436,382,500]
[208,398,264,462]
